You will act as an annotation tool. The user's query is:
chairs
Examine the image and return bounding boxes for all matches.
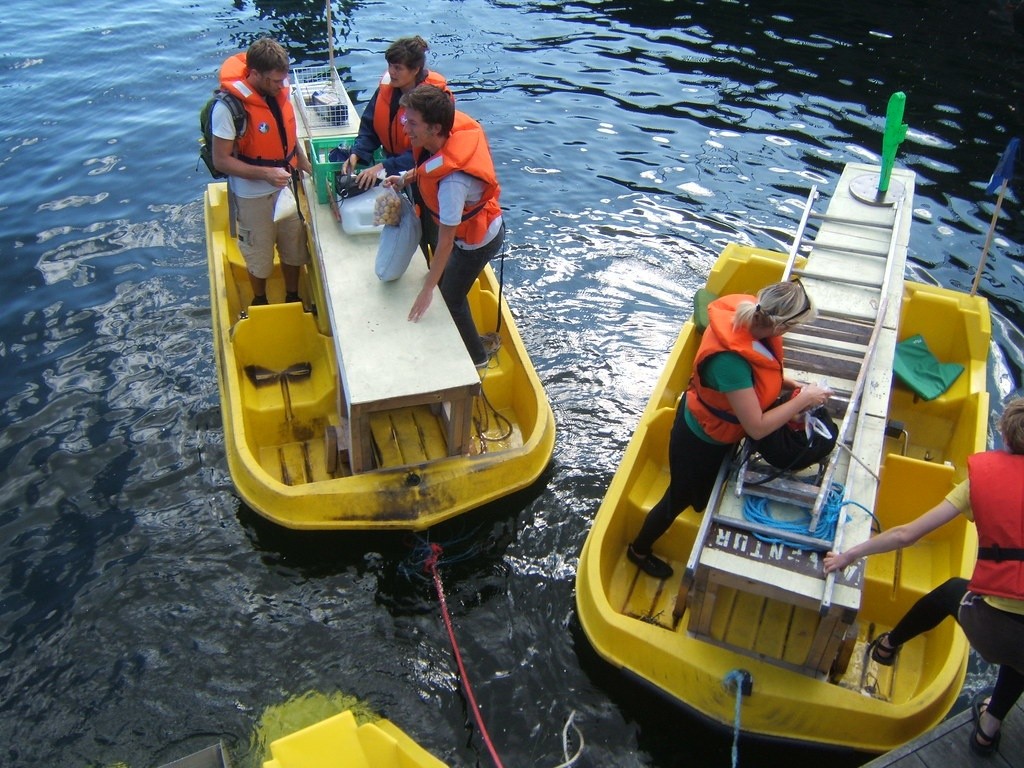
[622,406,727,562]
[210,190,304,315]
[888,290,982,427]
[231,302,339,448]
[717,255,786,296]
[857,452,957,637]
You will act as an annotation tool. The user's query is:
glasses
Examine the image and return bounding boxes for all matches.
[782,277,811,324]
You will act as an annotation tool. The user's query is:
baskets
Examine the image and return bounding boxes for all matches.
[293,67,350,129]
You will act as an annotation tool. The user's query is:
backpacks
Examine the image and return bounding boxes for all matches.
[199,89,247,180]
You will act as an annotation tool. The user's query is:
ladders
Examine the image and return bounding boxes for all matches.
[687,184,905,617]
[735,299,887,532]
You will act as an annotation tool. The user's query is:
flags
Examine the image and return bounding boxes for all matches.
[984,137,1018,195]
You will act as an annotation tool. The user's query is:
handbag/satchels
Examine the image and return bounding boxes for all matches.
[336,170,381,199]
[736,386,839,487]
[375,191,422,282]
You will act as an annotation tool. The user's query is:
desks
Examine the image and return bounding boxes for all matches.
[289,80,361,140]
[296,134,482,475]
[687,161,914,683]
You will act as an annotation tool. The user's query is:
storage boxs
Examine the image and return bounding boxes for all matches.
[308,136,387,206]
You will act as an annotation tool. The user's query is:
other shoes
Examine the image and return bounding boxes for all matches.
[626,544,674,579]
[286,298,312,313]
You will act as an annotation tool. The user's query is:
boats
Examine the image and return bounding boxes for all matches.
[201,0,556,534]
[567,85,994,754]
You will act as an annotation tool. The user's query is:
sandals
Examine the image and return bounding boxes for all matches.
[972,694,1001,757]
[872,628,902,665]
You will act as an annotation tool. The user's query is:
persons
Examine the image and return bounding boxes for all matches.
[340,37,456,268]
[212,38,311,311]
[823,396,1024,755]
[382,85,508,367]
[627,280,835,578]
